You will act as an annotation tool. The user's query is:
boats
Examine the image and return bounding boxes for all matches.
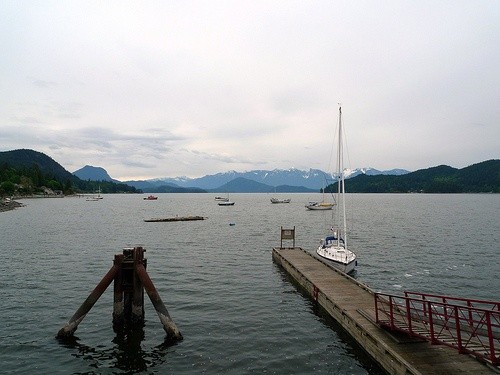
[214,195,235,206]
[143,195,158,200]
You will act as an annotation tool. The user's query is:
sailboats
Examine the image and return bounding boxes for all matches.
[305,171,338,211]
[270,184,292,203]
[85,185,103,202]
[316,106,356,274]
